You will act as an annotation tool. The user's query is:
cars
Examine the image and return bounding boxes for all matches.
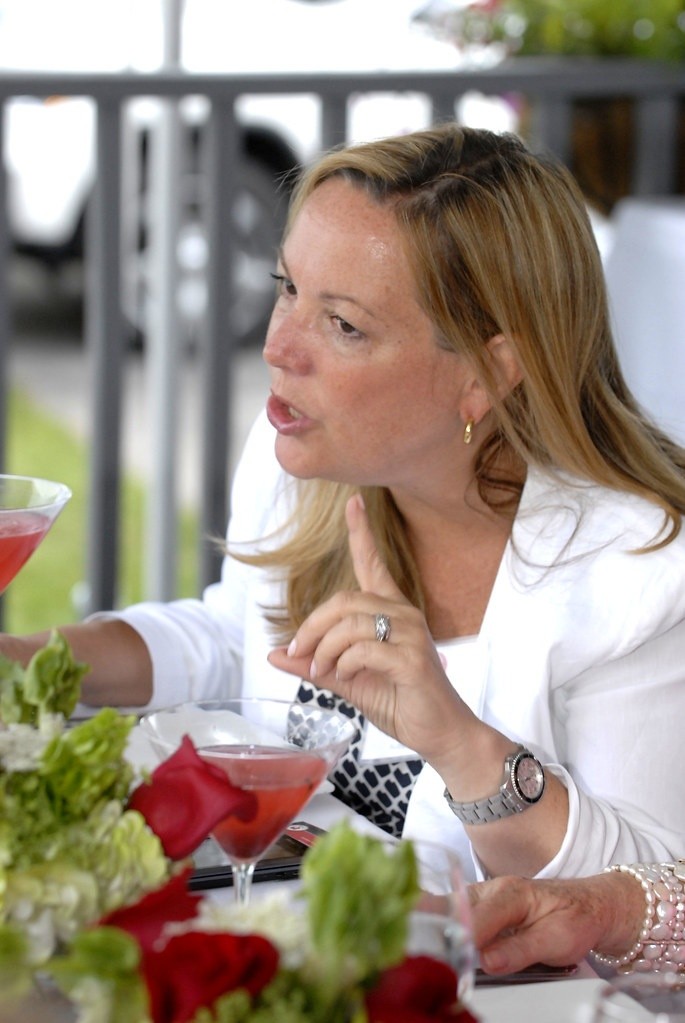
[0,0,531,359]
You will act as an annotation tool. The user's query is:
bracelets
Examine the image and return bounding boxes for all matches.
[588,861,685,992]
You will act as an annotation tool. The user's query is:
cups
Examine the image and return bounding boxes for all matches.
[0,474,75,599]
[381,839,476,1007]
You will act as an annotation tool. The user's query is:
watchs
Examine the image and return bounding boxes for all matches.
[444,744,546,826]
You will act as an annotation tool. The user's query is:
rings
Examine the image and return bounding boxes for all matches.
[375,614,391,640]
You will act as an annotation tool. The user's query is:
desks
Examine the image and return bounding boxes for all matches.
[68,707,654,1023]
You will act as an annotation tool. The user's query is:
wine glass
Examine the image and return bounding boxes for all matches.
[137,699,358,917]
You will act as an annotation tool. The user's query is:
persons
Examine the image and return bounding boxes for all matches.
[0,128,685,882]
[410,859,685,994]
